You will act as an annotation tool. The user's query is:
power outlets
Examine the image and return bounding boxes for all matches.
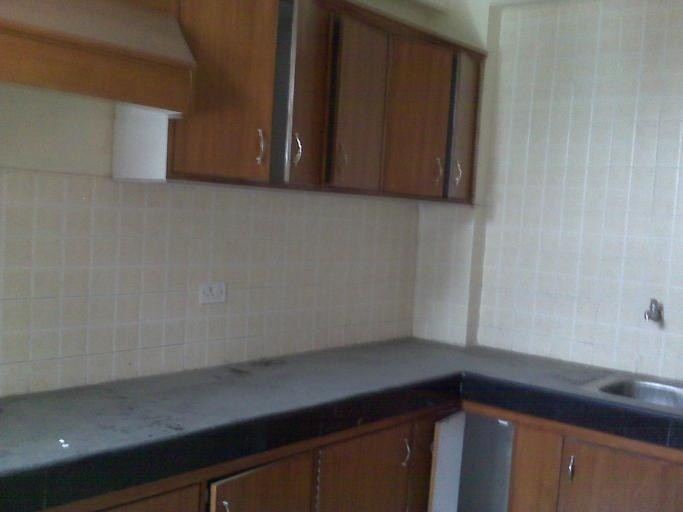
[198,281,226,305]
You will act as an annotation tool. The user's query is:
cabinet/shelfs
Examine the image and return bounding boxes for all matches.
[49,438,314,512]
[328,0,385,199]
[389,16,490,206]
[463,400,683,512]
[315,402,463,511]
[166,0,338,188]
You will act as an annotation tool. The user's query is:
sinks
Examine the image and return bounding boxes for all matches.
[598,378,683,410]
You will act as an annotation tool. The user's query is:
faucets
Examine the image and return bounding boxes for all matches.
[644,298,664,324]
